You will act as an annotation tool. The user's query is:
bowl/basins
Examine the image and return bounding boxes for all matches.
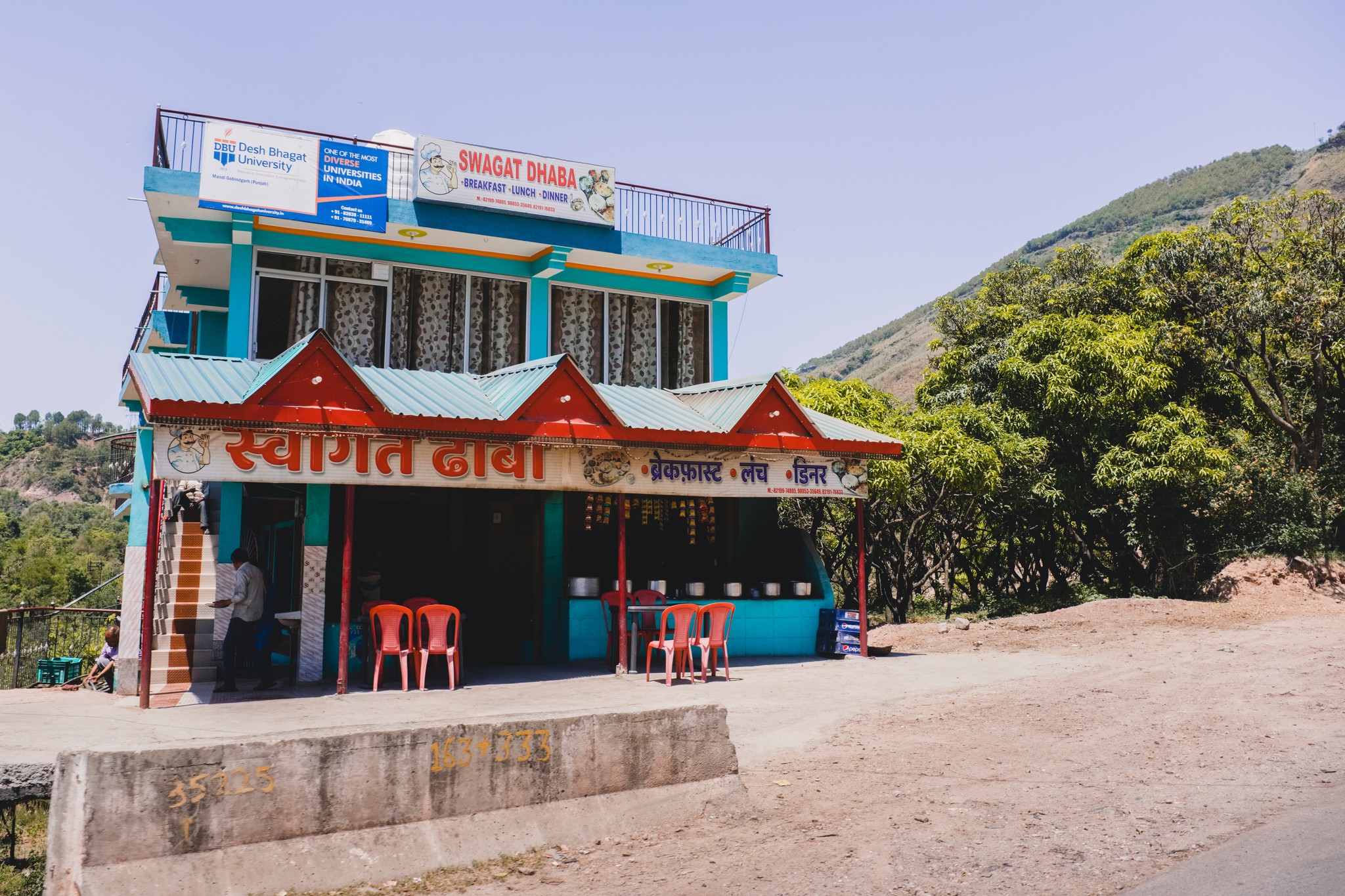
[593,182,613,199]
[606,196,614,207]
[589,194,606,212]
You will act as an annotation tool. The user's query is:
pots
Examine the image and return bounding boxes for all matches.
[790,581,812,596]
[748,588,761,597]
[723,582,742,598]
[564,576,601,599]
[760,582,781,598]
[668,588,683,598]
[610,579,633,594]
[684,582,707,597]
[647,579,667,595]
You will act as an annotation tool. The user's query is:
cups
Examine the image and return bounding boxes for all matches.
[588,169,602,184]
[601,170,613,184]
[579,176,594,193]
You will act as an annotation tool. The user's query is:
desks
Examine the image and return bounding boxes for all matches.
[352,613,467,689]
[607,604,710,672]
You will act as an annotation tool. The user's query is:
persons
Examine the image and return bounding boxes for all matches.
[164,480,212,535]
[213,547,274,692]
[82,625,142,693]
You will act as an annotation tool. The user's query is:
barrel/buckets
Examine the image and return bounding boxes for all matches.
[112,659,141,694]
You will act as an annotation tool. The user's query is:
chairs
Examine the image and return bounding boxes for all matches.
[601,589,736,686]
[363,597,461,692]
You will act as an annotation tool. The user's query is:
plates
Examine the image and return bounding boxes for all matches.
[570,198,584,211]
[205,601,219,606]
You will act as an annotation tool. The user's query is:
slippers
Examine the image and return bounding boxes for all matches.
[252,682,278,691]
[213,685,239,693]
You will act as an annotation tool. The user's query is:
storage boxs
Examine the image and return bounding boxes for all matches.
[356,568,381,602]
[36,657,83,684]
[816,609,861,655]
